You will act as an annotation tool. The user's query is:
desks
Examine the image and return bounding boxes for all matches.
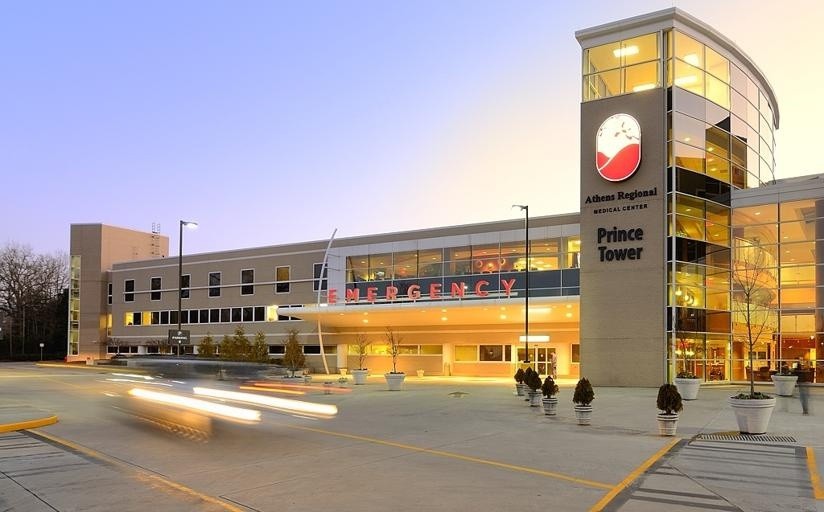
[750,369,777,381]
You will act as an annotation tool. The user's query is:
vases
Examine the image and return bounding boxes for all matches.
[770,373,798,396]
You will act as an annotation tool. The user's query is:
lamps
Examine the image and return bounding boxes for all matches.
[675,286,697,306]
[675,346,694,356]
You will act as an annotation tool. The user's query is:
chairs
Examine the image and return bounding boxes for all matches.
[757,366,769,381]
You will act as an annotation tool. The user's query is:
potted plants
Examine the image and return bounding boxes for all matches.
[729,391,776,434]
[673,371,701,400]
[350,369,369,385]
[417,369,424,377]
[655,383,683,436]
[572,377,593,425]
[514,366,559,416]
[383,371,407,391]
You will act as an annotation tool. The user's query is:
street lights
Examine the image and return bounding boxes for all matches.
[178,221,197,330]
[512,205,529,362]
